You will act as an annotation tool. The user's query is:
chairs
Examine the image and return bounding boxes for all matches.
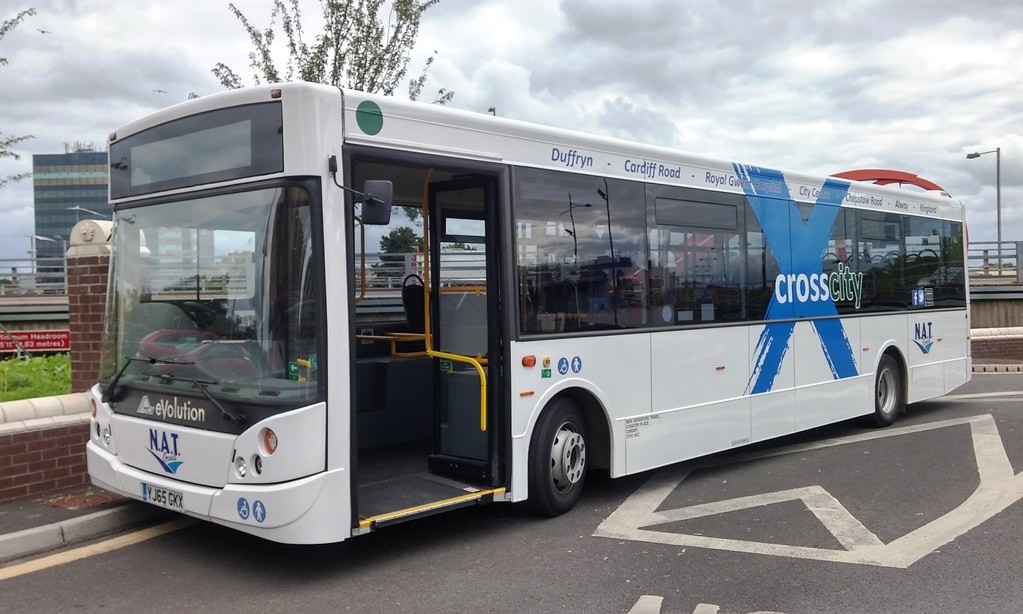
[821,248,942,318]
[401,273,426,334]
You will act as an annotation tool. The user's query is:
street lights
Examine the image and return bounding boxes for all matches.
[65,204,107,225]
[53,234,70,295]
[966,146,1002,276]
[24,231,56,274]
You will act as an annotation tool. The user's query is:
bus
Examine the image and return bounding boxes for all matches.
[85,80,972,546]
